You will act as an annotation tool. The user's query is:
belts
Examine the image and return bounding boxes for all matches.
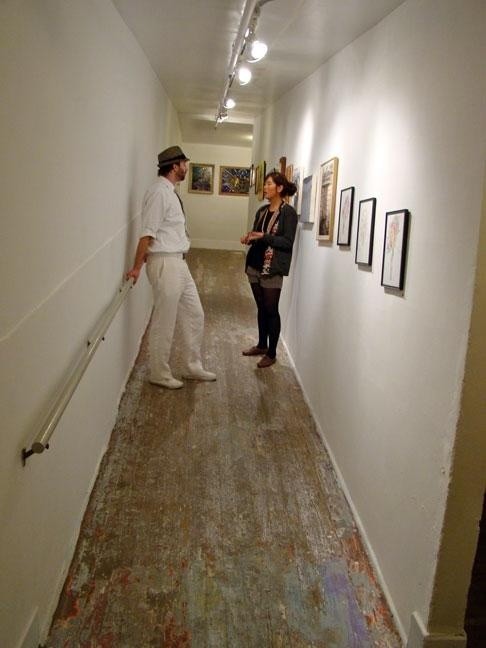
[149,252,186,259]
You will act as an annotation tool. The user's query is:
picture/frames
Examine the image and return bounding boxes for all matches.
[254,156,410,291]
[187,161,250,196]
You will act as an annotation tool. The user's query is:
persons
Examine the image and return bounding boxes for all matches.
[239,170,299,368]
[124,144,217,388]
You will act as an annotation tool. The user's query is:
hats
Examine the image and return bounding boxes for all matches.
[156,146,193,167]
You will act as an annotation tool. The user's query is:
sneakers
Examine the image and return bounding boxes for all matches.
[183,369,217,381]
[148,375,184,390]
[242,346,268,355]
[257,354,277,368]
